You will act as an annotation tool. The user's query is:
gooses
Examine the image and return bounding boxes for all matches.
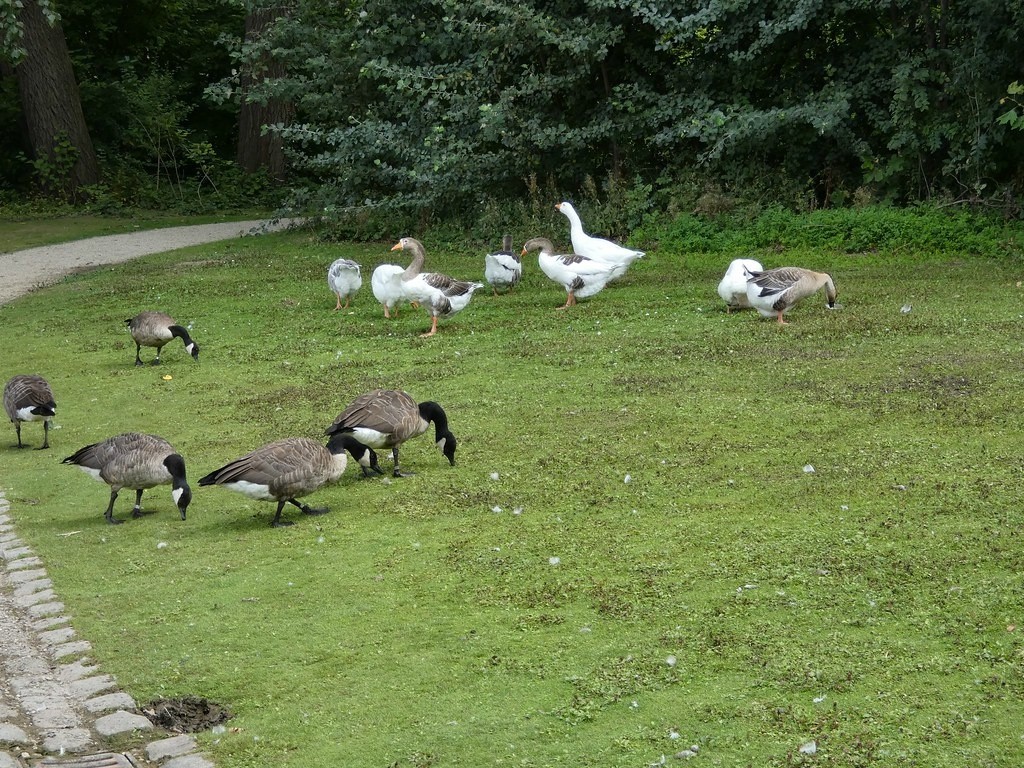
[742,264,836,325]
[718,259,763,313]
[484,235,522,297]
[555,202,646,289]
[327,258,362,311]
[371,264,418,318]
[520,238,625,309]
[390,237,484,338]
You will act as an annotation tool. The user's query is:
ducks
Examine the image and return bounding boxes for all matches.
[124,311,200,366]
[324,389,456,477]
[60,432,192,525]
[3,375,58,451]
[197,433,386,528]
